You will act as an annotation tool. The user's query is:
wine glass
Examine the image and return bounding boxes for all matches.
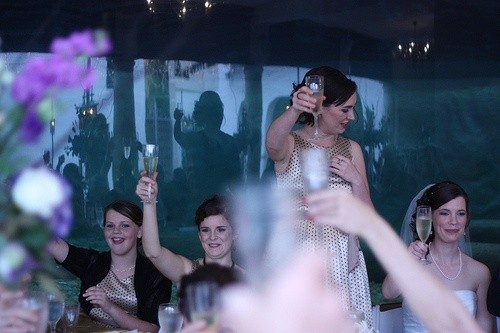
[416,205,433,266]
[47,293,65,333]
[304,74,327,142]
[140,143,159,204]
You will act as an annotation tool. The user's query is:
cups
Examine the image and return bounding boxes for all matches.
[158,302,183,333]
[186,280,219,330]
[15,291,81,333]
[299,149,329,194]
[225,180,296,286]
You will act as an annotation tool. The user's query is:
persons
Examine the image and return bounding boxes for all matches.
[136,171,250,304]
[0,273,38,333]
[267,69,372,333]
[383,183,498,333]
[180,190,486,333]
[42,201,172,333]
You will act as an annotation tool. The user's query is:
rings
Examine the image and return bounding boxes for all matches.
[297,92,302,99]
[337,159,343,164]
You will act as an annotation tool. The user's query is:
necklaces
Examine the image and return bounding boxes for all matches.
[110,263,135,271]
[303,125,338,147]
[429,242,462,280]
[203,255,234,269]
